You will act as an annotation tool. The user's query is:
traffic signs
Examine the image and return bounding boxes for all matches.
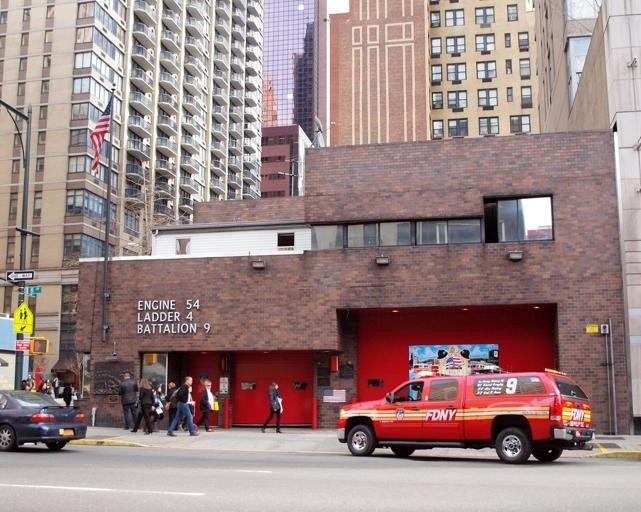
[15,286,42,298]
[4,270,37,282]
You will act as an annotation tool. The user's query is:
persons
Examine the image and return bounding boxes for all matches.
[143,381,163,432]
[179,386,193,433]
[118,372,138,429]
[23,375,74,407]
[167,377,200,437]
[259,382,283,433]
[195,380,215,433]
[165,382,179,431]
[130,378,156,436]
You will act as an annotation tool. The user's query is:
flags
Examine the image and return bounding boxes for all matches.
[92,82,118,178]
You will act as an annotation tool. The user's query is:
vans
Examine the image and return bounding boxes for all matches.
[336,365,598,465]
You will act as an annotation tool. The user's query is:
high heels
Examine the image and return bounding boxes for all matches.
[261,427,266,434]
[276,429,281,433]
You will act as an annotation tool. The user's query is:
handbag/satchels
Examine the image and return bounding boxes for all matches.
[271,400,280,411]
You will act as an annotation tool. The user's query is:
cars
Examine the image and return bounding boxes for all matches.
[0,390,88,451]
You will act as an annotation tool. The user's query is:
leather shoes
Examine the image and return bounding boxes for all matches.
[207,429,214,432]
[167,431,175,437]
[188,430,200,437]
[126,425,159,435]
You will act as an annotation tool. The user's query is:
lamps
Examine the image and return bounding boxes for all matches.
[507,251,523,261]
[112,341,117,356]
[250,261,265,269]
[375,256,390,265]
